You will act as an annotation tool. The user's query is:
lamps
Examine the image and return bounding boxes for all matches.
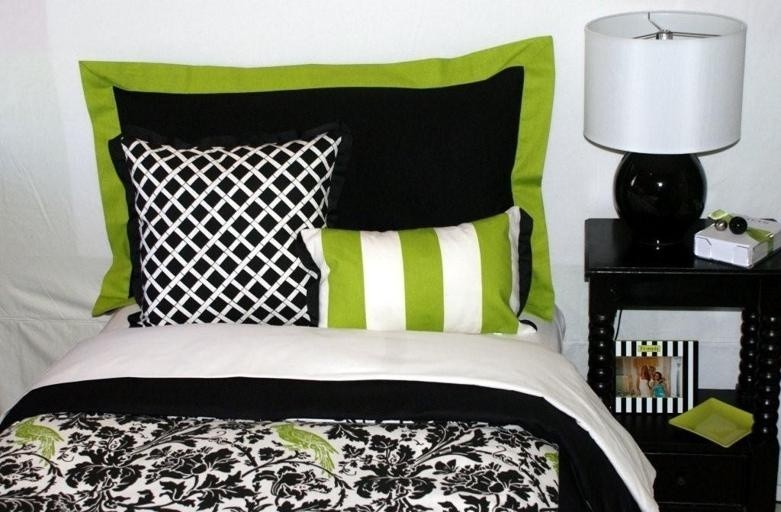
[582,7,747,253]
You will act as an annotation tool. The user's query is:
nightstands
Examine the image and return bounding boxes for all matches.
[580,217,780,507]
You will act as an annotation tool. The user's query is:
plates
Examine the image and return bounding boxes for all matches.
[668,397,755,449]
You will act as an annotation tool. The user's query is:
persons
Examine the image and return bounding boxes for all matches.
[636,364,669,398]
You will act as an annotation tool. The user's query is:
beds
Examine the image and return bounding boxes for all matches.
[0,252,659,512]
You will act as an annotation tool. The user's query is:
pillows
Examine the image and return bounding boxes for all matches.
[118,133,342,326]
[301,207,540,336]
[79,33,555,323]
